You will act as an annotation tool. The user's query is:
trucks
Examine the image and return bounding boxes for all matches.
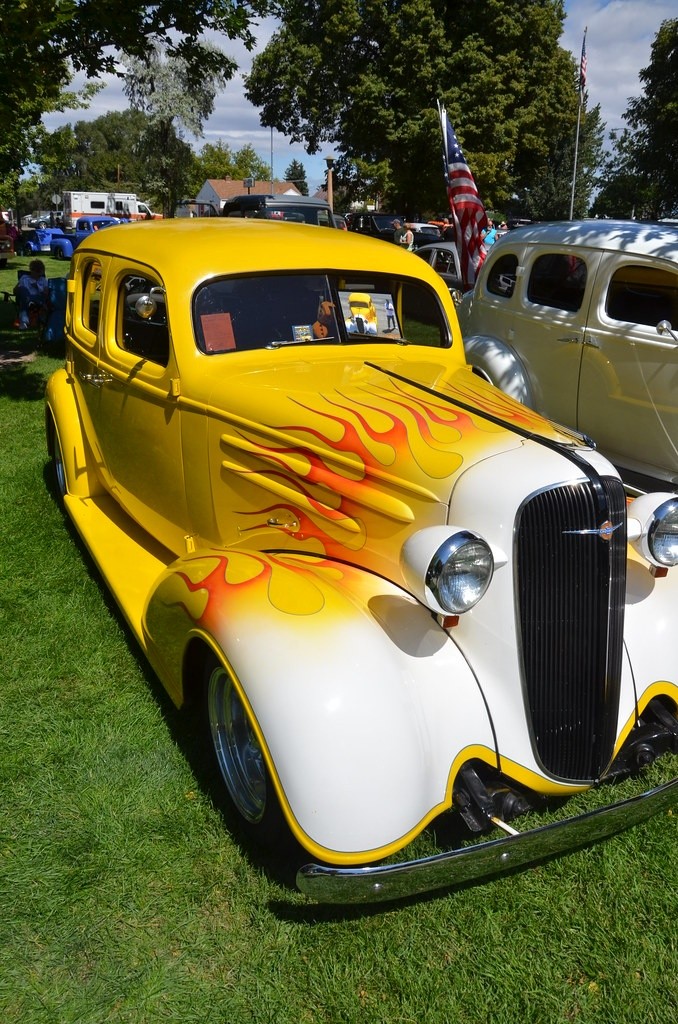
[63,191,165,229]
[50,217,120,261]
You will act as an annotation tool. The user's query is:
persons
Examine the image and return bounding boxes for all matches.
[444,217,507,252]
[390,219,414,253]
[12,259,48,331]
[384,299,398,330]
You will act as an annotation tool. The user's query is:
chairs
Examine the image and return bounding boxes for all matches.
[6,271,67,354]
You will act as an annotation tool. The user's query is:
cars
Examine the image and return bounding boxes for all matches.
[45,215,678,904]
[0,219,17,268]
[345,293,378,334]
[28,211,63,230]
[18,227,64,257]
[283,212,454,251]
[447,219,678,495]
[170,194,337,229]
[412,242,493,294]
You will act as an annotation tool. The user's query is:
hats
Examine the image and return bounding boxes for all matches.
[390,219,401,224]
[501,221,506,225]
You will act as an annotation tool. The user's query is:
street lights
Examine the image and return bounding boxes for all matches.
[323,156,337,213]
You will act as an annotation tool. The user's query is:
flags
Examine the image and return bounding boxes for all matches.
[441,108,488,292]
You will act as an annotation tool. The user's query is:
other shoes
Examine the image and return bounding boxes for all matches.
[18,311,31,330]
